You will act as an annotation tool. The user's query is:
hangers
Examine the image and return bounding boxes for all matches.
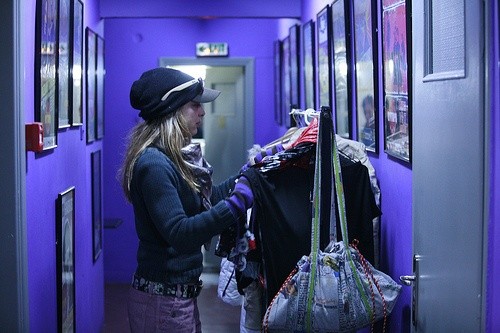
[276,108,317,148]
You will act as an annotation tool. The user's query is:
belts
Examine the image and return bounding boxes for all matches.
[132,275,204,298]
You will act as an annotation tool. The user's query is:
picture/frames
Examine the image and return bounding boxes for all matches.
[316,5,332,109]
[281,36,296,129]
[353,0,379,154]
[273,40,282,126]
[330,0,352,140]
[288,24,301,109]
[91,150,103,263]
[381,0,412,166]
[57,185,76,333]
[303,20,316,111]
[35,0,105,153]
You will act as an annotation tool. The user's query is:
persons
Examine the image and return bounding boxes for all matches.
[124,65,254,333]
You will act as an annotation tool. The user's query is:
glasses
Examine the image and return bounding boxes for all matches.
[161,77,203,101]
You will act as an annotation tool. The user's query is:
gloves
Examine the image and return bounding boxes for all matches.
[237,160,251,174]
[224,176,254,215]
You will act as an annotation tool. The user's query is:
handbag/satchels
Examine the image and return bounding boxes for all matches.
[262,114,402,333]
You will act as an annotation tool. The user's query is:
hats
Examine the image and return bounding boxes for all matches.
[130,67,221,125]
